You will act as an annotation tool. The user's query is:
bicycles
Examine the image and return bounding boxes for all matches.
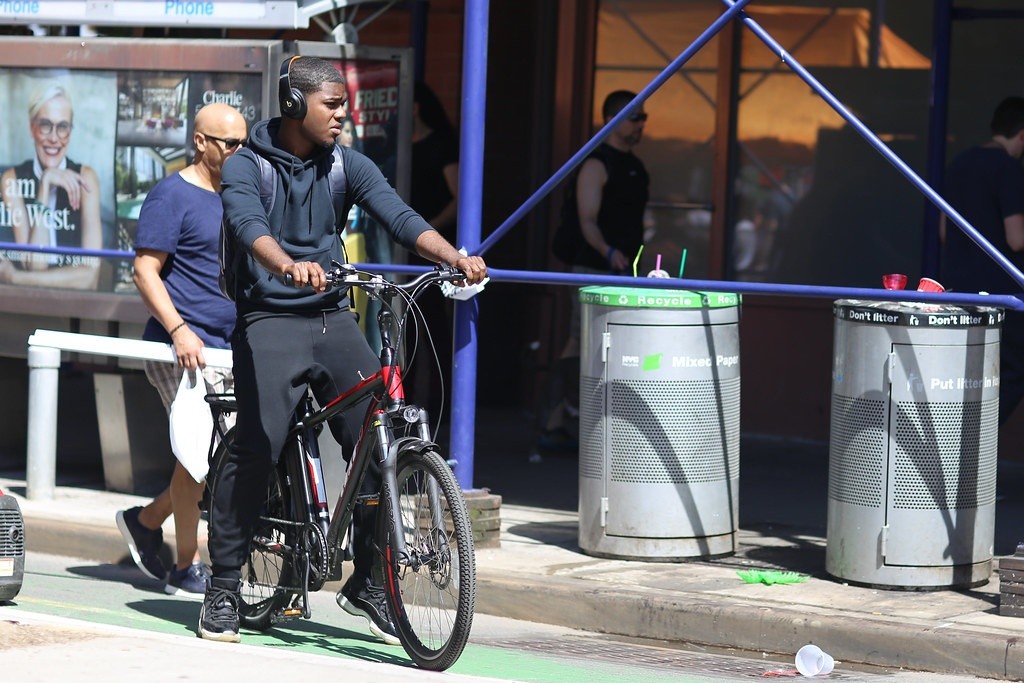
[197,260,490,672]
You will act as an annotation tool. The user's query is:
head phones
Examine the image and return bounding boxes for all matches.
[279,55,307,119]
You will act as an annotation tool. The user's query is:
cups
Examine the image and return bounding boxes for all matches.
[883,274,907,290]
[917,277,945,292]
[646,269,670,278]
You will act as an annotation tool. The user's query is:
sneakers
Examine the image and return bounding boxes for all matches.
[165,561,215,602]
[115,506,167,581]
[336,568,402,647]
[199,577,242,645]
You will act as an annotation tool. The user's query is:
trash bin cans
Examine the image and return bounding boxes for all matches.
[578,284,745,560]
[825,300,1002,590]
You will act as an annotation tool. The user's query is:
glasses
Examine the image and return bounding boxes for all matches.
[628,113,647,122]
[39,119,71,139]
[204,134,248,151]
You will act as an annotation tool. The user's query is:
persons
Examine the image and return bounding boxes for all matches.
[197,55,488,644]
[393,79,458,494]
[531,89,651,456]
[0,80,102,290]
[116,103,249,600]
[939,93,1024,502]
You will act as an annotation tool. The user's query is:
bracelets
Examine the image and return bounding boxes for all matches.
[607,247,614,268]
[170,322,185,336]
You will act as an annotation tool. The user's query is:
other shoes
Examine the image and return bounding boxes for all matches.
[536,425,579,460]
